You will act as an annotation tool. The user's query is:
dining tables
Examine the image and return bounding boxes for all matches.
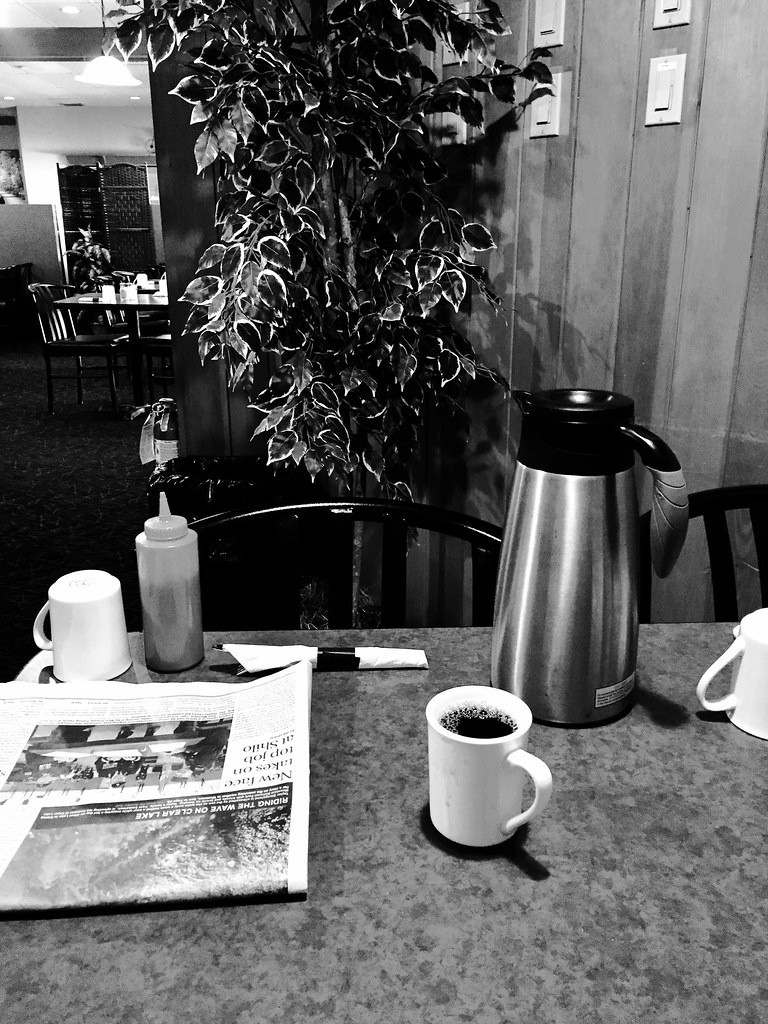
[53,293,169,413]
[2,631,767,1024]
[135,284,160,292]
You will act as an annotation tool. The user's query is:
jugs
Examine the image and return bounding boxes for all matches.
[486,386,690,730]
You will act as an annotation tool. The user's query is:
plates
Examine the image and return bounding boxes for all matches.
[152,291,168,297]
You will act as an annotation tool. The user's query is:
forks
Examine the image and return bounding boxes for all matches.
[235,661,428,677]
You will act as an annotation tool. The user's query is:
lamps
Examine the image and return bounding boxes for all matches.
[72,1,143,87]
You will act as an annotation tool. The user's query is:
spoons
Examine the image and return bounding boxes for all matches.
[210,642,423,658]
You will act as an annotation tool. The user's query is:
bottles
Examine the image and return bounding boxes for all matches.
[133,490,206,676]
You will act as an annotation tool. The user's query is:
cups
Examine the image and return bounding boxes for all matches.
[119,282,132,300]
[422,684,553,850]
[132,273,147,287]
[32,568,134,683]
[695,607,768,740]
[102,285,115,304]
[158,280,167,291]
[141,281,154,288]
[161,271,166,280]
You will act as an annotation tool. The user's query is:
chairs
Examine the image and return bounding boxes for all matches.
[187,498,505,631]
[640,484,768,624]
[0,262,176,417]
